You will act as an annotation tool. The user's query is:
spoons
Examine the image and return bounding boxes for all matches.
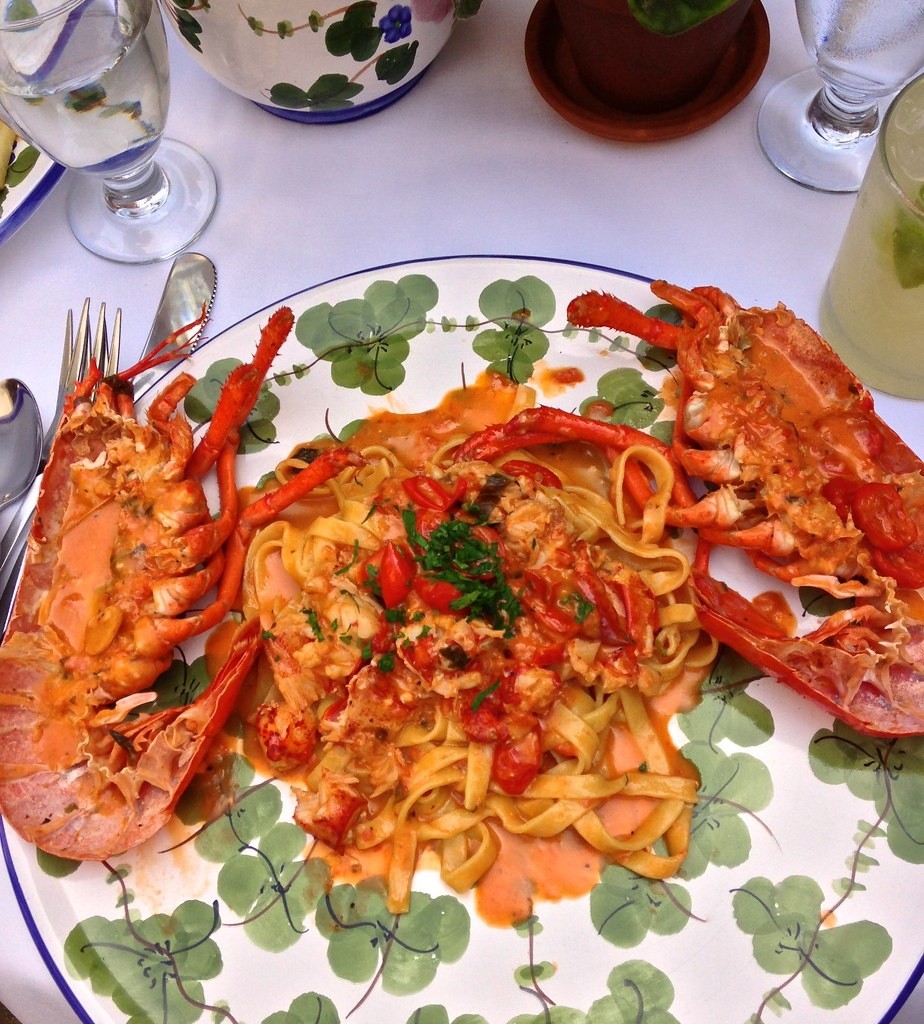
[0,374,46,514]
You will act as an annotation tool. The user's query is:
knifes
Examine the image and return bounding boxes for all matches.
[140,248,223,377]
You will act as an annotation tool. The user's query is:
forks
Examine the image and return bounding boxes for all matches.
[0,293,127,642]
[0,307,116,578]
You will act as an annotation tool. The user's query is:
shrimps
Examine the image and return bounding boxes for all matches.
[452,279,924,735]
[2,304,371,864]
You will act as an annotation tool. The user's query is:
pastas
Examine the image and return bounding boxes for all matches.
[224,399,717,914]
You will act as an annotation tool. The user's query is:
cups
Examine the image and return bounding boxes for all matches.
[814,68,923,407]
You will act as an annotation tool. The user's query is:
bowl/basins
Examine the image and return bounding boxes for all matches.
[517,0,770,140]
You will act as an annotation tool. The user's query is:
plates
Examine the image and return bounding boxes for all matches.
[3,255,922,1024]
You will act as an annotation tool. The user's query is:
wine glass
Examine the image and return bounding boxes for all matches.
[1,0,218,266]
[751,1,924,201]
